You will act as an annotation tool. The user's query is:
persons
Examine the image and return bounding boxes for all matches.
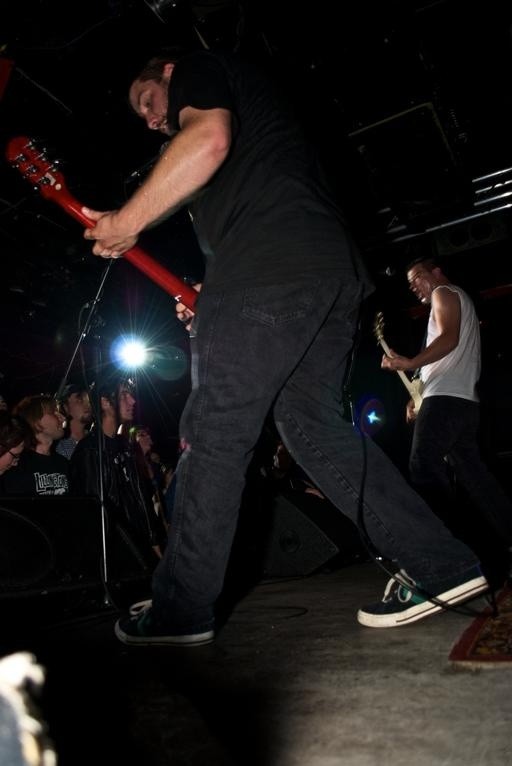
[221,436,329,578]
[377,255,504,582]
[82,45,489,650]
[0,374,189,594]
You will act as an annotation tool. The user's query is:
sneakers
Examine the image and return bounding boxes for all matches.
[357,566,490,631]
[113,600,217,648]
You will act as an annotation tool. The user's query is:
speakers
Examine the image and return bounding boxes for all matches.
[254,494,360,578]
[0,493,150,603]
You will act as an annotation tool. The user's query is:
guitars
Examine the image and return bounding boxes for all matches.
[374,312,423,416]
[8,138,199,313]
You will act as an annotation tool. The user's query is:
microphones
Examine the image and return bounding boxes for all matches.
[377,266,396,277]
[129,141,170,179]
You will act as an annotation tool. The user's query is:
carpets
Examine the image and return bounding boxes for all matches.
[448,588,512,671]
